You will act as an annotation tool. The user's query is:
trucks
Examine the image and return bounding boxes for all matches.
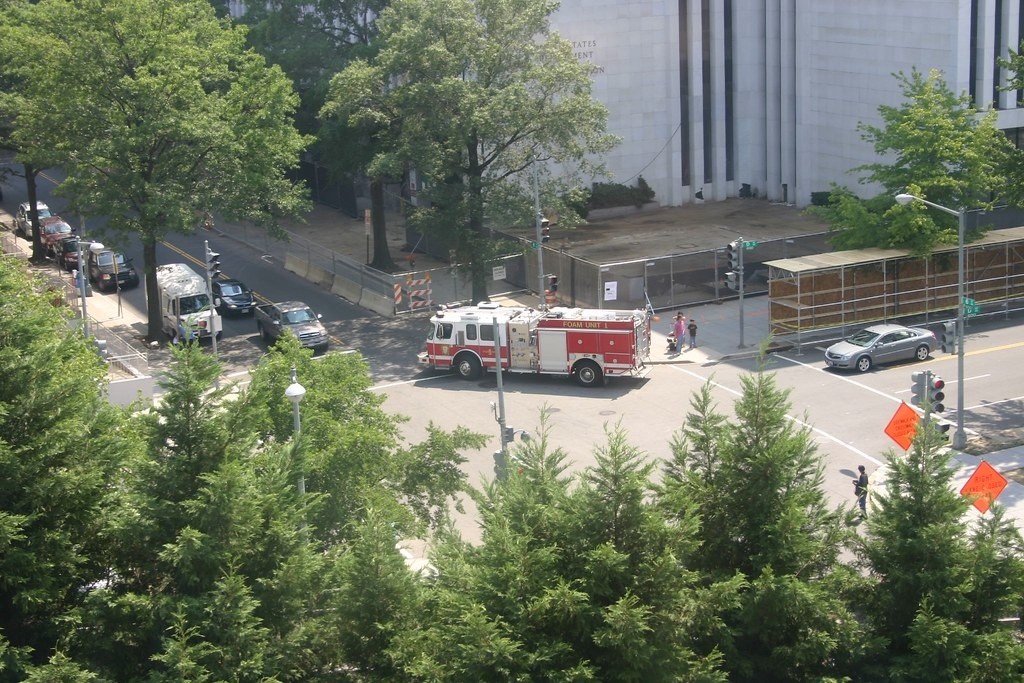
[145,263,222,347]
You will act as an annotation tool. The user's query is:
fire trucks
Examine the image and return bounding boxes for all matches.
[417,302,654,388]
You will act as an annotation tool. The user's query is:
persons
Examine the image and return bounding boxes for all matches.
[672,312,697,355]
[853,465,868,520]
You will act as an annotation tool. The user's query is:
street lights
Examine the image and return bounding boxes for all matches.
[894,192,967,451]
[283,362,311,551]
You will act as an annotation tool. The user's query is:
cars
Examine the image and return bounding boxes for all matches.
[45,234,92,271]
[212,279,257,319]
[17,201,57,237]
[824,324,938,374]
[38,217,77,247]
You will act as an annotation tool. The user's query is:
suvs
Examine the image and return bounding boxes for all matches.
[89,248,139,294]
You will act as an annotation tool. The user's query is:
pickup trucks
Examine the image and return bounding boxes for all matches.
[254,301,329,354]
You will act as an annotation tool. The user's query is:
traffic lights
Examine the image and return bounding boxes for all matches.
[910,372,925,407]
[547,275,559,293]
[935,421,951,441]
[724,271,735,290]
[205,252,222,281]
[726,240,738,272]
[941,320,956,355]
[539,219,552,243]
[928,372,945,414]
